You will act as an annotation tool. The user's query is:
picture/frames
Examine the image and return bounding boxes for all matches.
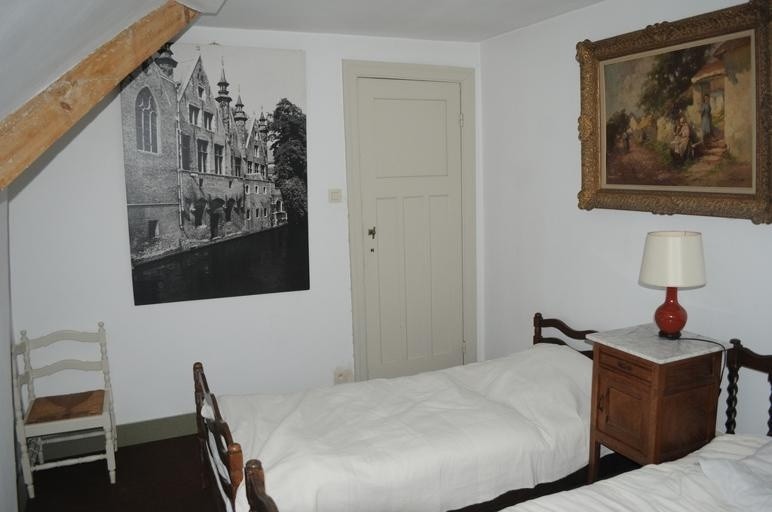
[576,0,772,223]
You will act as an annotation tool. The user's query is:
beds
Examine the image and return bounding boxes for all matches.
[194,312,632,511]
[496,346,771,511]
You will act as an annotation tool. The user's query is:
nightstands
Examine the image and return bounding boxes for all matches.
[586,318,722,484]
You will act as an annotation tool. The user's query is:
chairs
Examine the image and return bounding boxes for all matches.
[6,326,123,500]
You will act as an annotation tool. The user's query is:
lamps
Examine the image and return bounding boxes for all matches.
[635,231,703,339]
